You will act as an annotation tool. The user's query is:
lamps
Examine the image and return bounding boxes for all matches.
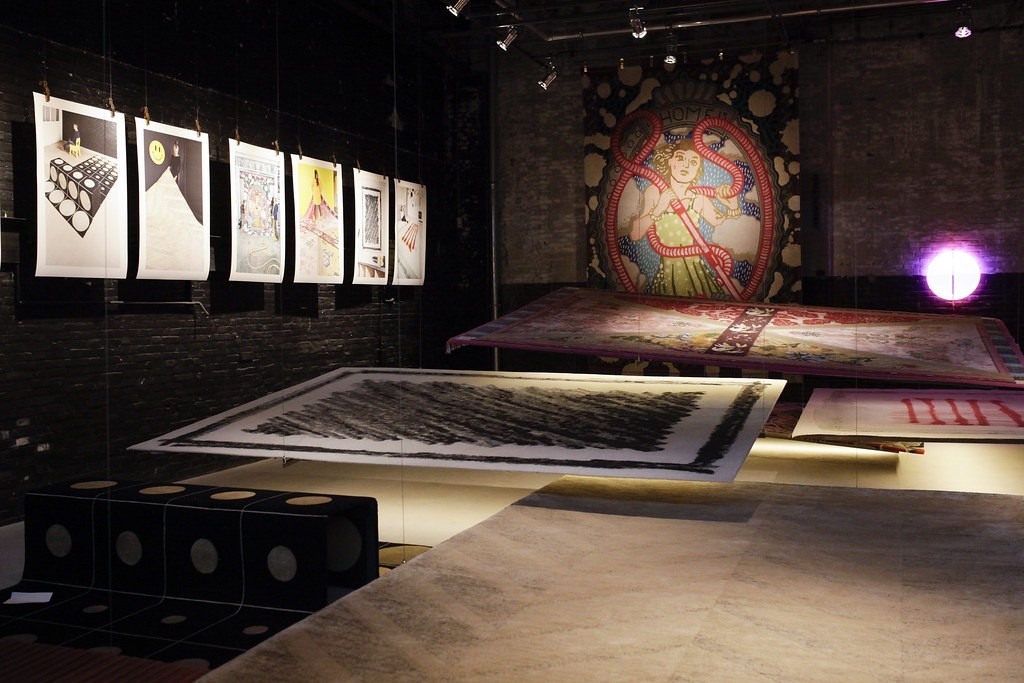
[664,28,678,64]
[539,61,558,90]
[500,27,518,52]
[631,6,647,39]
[447,0,469,16]
